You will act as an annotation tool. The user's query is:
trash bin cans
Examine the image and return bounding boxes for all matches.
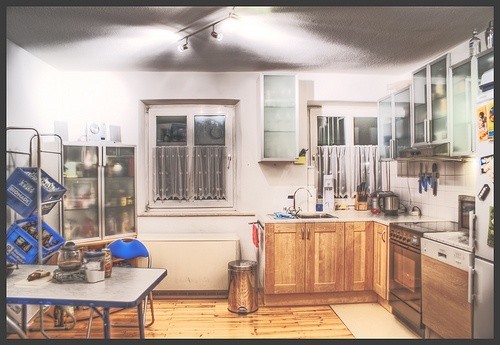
[228,260,258,314]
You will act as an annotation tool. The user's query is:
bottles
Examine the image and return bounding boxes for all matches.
[85,248,113,282]
[13,221,51,253]
[469,20,494,54]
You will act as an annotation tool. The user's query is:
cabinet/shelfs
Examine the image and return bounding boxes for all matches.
[375,82,414,163]
[6,127,65,339]
[448,54,478,160]
[44,235,140,323]
[32,142,137,244]
[258,73,300,163]
[412,52,465,146]
[256,221,345,307]
[373,221,392,314]
[344,222,379,303]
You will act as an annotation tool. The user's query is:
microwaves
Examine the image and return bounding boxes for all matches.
[457,195,476,235]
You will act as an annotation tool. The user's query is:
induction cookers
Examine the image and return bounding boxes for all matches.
[390,221,458,250]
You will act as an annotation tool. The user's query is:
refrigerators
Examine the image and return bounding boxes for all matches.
[467,88,495,339]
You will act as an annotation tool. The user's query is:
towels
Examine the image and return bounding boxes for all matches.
[251,223,260,248]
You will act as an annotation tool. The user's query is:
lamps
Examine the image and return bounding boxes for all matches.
[210,25,223,40]
[178,36,189,52]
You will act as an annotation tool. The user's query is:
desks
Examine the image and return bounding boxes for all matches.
[5,262,168,340]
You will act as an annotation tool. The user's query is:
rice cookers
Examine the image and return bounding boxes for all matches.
[369,191,393,212]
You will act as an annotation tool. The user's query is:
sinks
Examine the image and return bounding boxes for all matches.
[297,213,340,219]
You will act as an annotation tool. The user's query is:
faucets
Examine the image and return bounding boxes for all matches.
[293,187,313,216]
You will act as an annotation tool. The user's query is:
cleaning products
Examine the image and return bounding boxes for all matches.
[316,191,323,211]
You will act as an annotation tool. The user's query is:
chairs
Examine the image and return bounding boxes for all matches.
[93,237,156,329]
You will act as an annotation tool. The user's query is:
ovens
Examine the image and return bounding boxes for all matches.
[389,239,426,338]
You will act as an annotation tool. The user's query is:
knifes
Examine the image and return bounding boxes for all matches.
[357,182,369,202]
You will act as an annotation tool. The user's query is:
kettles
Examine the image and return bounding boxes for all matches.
[378,193,399,216]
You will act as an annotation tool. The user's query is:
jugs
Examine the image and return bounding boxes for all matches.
[57,240,88,272]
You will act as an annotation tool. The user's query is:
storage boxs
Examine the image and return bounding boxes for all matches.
[6,166,68,218]
[355,191,368,212]
[6,215,66,265]
